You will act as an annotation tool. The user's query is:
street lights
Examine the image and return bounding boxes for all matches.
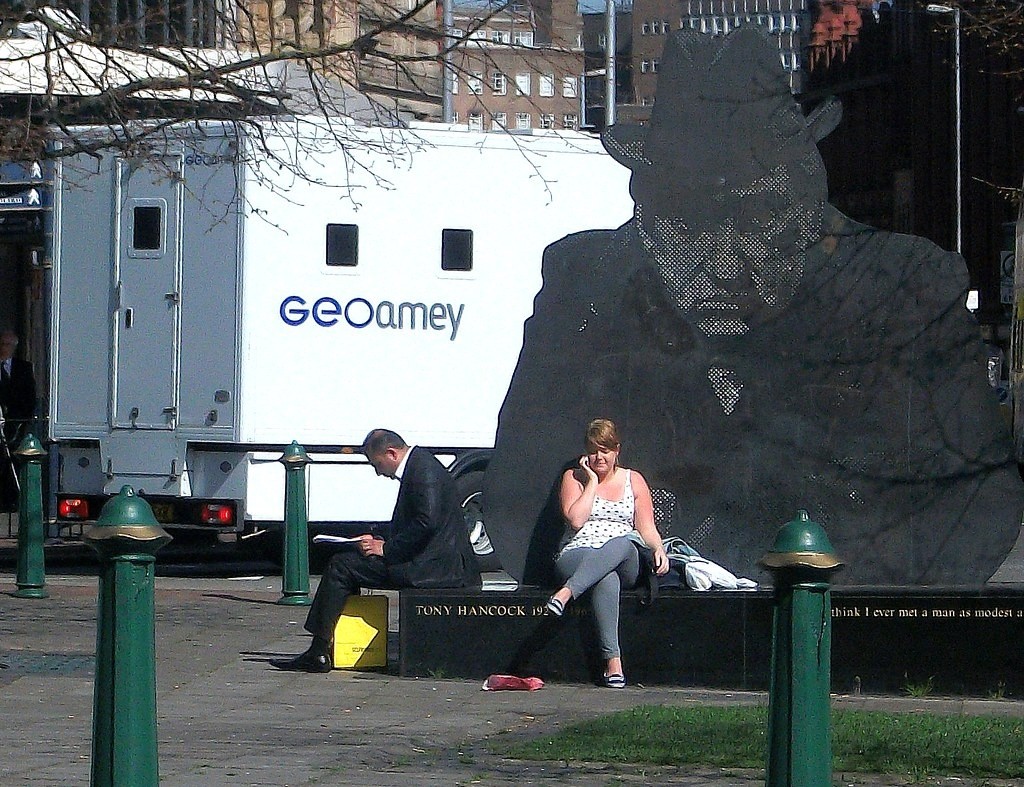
[927,5,961,254]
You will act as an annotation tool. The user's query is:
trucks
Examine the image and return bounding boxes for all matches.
[47,113,635,572]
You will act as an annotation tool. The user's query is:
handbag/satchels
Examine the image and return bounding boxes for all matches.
[637,538,689,605]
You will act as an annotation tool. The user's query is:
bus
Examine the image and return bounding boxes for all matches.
[987,182,1024,479]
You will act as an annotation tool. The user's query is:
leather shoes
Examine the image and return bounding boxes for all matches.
[269,652,332,673]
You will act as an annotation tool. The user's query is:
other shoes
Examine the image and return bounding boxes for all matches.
[604,671,626,688]
[544,594,564,617]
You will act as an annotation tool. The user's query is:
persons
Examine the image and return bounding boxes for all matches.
[545,419,670,688]
[268,428,477,673]
[1,332,37,515]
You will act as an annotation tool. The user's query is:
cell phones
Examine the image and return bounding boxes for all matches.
[585,456,590,467]
